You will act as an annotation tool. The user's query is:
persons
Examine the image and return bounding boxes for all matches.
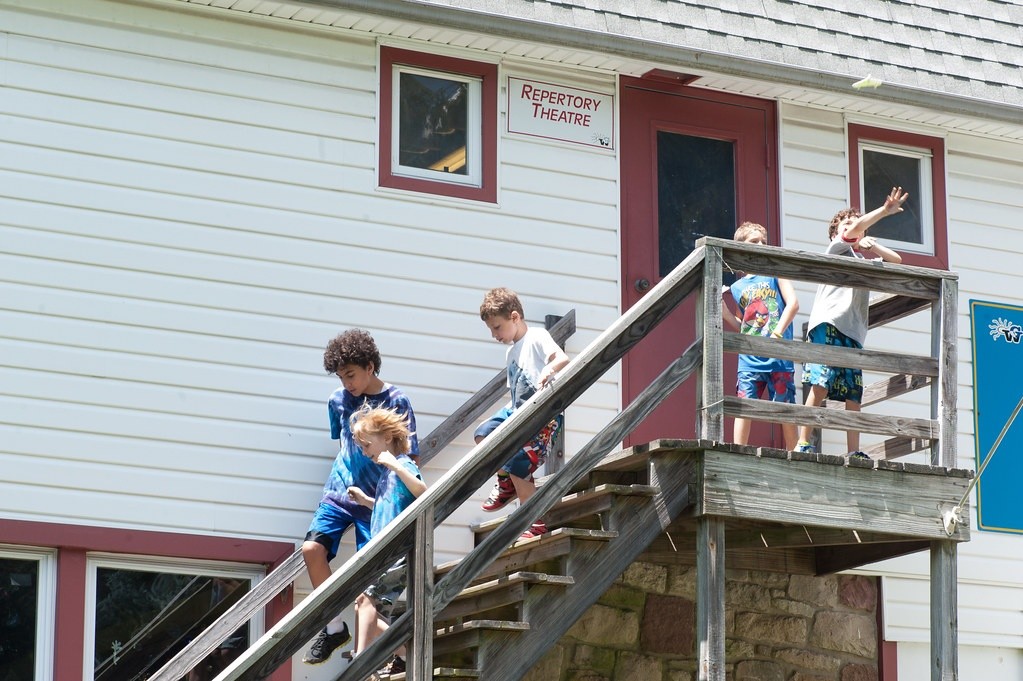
[302,328,419,664]
[347,408,427,681]
[721,222,799,451]
[473,288,570,540]
[793,187,909,459]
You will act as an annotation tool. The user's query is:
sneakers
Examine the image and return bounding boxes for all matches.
[302,621,351,665]
[513,520,550,542]
[482,477,518,512]
[792,442,816,454]
[376,653,407,679]
[847,451,871,460]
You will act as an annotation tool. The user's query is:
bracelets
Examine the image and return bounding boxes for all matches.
[774,332,782,338]
